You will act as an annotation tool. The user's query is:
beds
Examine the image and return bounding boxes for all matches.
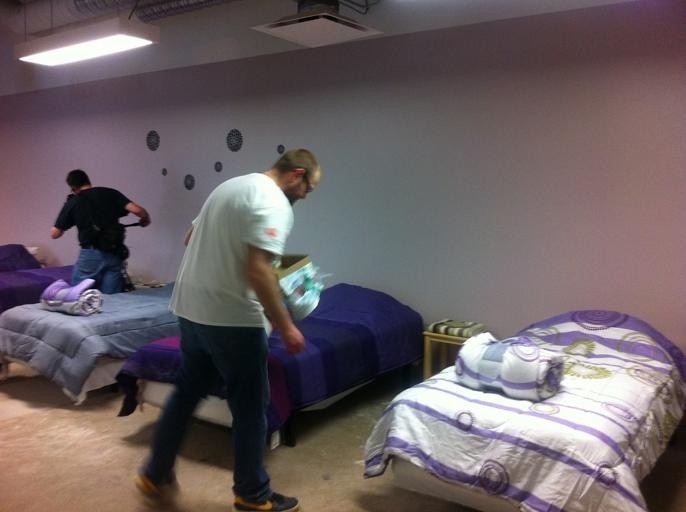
[0,277,180,404]
[1,256,89,316]
[362,310,685,511]
[116,282,424,450]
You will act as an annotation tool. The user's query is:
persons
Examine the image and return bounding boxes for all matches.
[132,148,320,512]
[49,170,151,294]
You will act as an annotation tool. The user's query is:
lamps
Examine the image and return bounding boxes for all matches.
[12,1,161,73]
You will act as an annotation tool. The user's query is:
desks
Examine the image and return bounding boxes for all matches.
[420,327,469,382]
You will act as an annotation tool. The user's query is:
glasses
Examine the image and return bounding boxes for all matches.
[304,177,314,193]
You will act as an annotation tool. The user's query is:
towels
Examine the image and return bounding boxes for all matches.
[428,318,489,341]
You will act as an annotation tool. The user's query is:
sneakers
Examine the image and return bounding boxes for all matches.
[233,491,300,512]
